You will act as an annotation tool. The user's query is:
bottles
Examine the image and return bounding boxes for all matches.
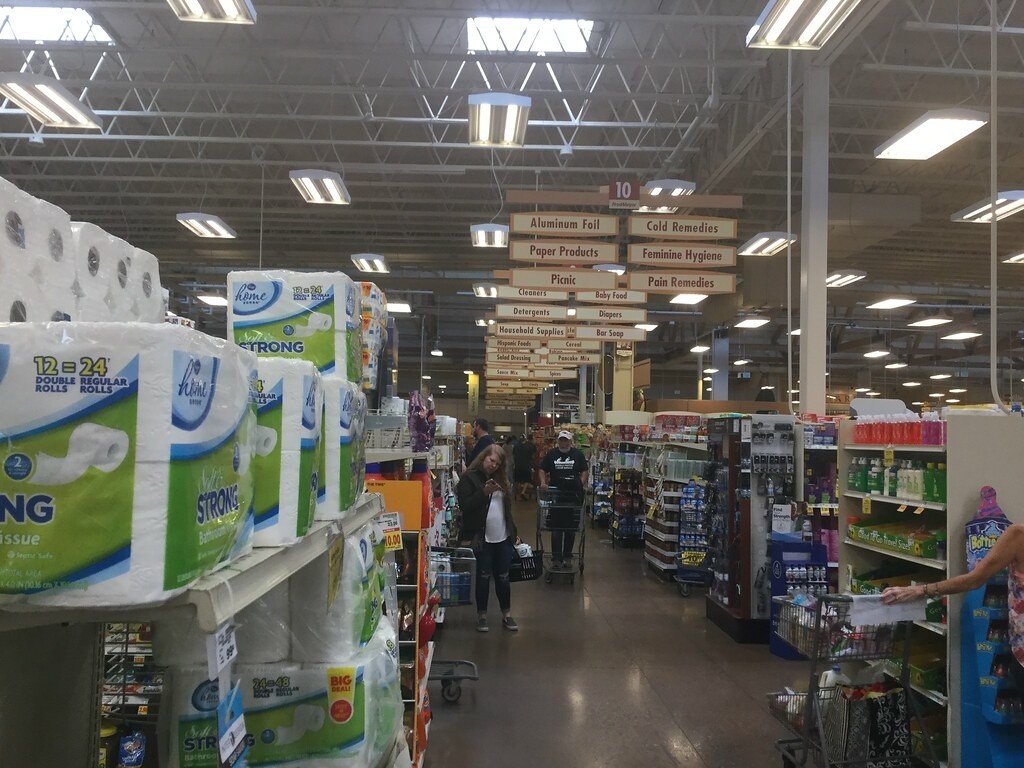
[848,456,946,504]
[819,666,845,717]
[851,411,947,445]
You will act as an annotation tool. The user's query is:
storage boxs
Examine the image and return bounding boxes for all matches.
[429,552,452,574]
[437,443,456,465]
[435,415,451,436]
[450,416,459,435]
[429,443,450,466]
[847,518,947,763]
[438,572,472,602]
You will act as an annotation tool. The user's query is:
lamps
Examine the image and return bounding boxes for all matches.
[667,293,709,306]
[864,293,919,312]
[905,315,954,329]
[950,190,1024,227]
[383,295,414,315]
[827,268,869,289]
[469,222,511,250]
[940,326,986,342]
[731,314,773,330]
[743,0,863,51]
[173,210,238,242]
[1000,249,1024,264]
[786,326,800,339]
[592,263,626,277]
[289,167,350,207]
[420,338,480,395]
[735,230,797,260]
[634,320,660,332]
[0,72,104,130]
[472,282,500,299]
[872,106,989,163]
[348,252,393,278]
[192,290,227,309]
[164,0,258,26]
[474,317,497,327]
[689,345,969,410]
[467,87,533,152]
[633,175,697,215]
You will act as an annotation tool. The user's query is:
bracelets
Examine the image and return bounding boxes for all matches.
[935,582,938,595]
[924,583,929,597]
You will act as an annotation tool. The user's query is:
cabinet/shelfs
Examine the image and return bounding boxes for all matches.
[837,407,1024,768]
[0,484,406,768]
[368,392,435,765]
[642,408,709,587]
[608,427,643,554]
[428,412,465,625]
[367,477,441,768]
[587,423,611,528]
[704,414,839,644]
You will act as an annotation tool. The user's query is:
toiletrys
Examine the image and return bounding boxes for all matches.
[852,409,948,447]
[846,455,948,503]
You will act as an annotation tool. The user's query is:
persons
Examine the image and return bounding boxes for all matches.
[538,430,588,568]
[882,522,1024,718]
[499,433,533,500]
[464,419,494,467]
[456,444,519,632]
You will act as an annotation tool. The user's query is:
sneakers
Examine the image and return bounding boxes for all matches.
[502,616,518,630]
[475,619,489,631]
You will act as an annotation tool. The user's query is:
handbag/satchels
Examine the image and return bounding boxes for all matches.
[821,680,912,768]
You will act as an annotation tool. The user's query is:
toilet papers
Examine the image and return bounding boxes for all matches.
[1,171,416,768]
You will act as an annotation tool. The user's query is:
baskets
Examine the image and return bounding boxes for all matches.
[508,544,544,582]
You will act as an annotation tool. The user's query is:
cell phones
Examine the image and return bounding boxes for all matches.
[487,479,497,485]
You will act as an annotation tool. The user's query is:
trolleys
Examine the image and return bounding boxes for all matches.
[425,544,478,702]
[533,485,587,585]
[769,589,938,768]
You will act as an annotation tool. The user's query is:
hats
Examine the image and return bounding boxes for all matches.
[558,430,573,440]
